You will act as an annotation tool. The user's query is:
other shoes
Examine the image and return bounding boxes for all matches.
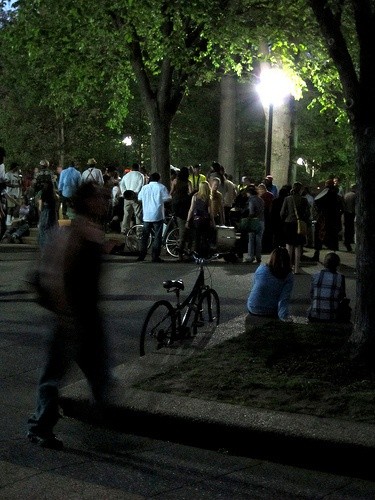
[152,258,164,262]
[12,235,20,243]
[111,436,147,450]
[138,252,147,260]
[28,431,63,448]
[257,259,261,262]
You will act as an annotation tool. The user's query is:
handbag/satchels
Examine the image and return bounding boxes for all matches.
[297,220,308,234]
[193,208,201,216]
[336,297,352,321]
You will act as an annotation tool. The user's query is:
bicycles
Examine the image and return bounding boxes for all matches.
[140,246,233,356]
[125,211,189,258]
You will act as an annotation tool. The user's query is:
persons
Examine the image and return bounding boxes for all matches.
[26,184,144,453]
[307,253,346,322]
[247,246,294,323]
[0,158,356,274]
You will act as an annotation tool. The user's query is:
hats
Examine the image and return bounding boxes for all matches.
[87,159,97,165]
[9,162,19,170]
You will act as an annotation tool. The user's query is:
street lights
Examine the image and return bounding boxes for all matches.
[254,66,292,178]
